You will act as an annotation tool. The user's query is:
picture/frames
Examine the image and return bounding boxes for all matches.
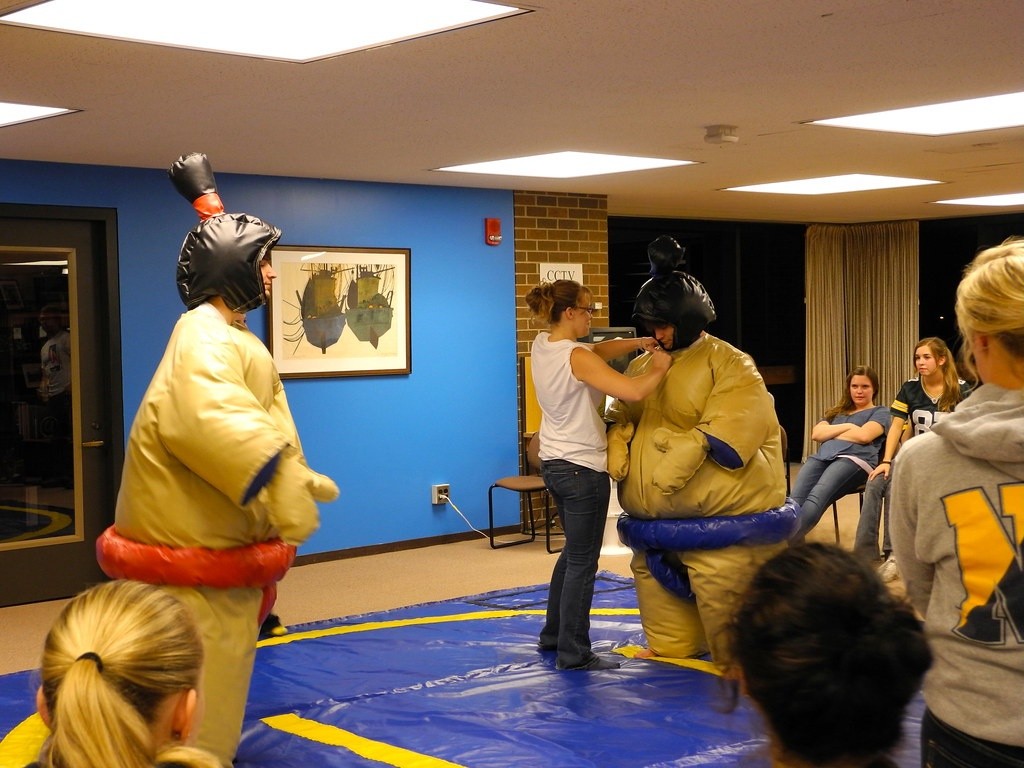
[266,243,412,379]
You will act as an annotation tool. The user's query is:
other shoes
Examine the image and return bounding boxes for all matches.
[41,476,74,489]
[878,552,901,582]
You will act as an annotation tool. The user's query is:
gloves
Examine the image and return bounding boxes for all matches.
[651,427,711,495]
[258,448,340,546]
[607,422,634,482]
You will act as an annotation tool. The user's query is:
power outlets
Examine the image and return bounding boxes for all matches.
[432,483,450,504]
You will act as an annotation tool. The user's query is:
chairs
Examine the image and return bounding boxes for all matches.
[780,424,791,498]
[487,432,567,554]
[831,439,887,541]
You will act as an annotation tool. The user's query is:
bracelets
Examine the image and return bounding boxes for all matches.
[640,337,645,352]
[880,460,891,464]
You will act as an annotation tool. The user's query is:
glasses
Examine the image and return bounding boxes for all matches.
[565,305,592,316]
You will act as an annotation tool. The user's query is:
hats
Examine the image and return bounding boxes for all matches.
[632,236,716,352]
[167,153,282,314]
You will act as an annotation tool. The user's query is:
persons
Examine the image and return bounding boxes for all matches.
[729,544,934,768]
[790,365,891,544]
[24,581,226,768]
[95,151,338,768]
[608,271,787,672]
[525,280,672,670]
[33,306,73,489]
[886,240,1024,767]
[853,338,973,567]
